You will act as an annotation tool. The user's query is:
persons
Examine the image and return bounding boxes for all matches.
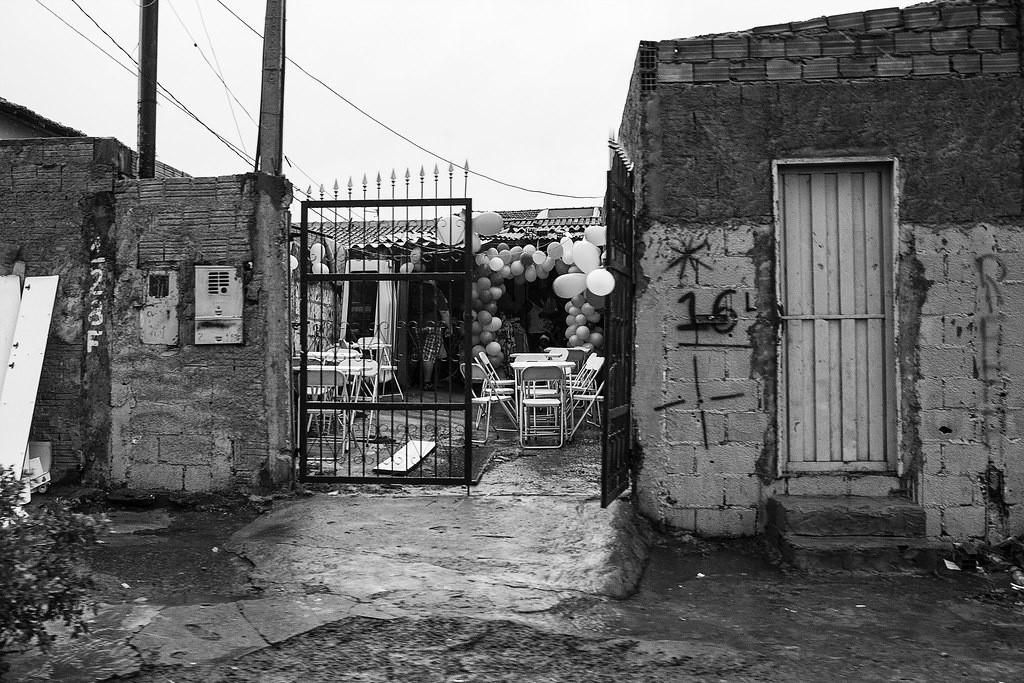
[535,335,549,353]
[518,279,565,353]
[413,271,450,390]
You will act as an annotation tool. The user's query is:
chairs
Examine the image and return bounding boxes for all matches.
[459,346,606,449]
[289,337,404,462]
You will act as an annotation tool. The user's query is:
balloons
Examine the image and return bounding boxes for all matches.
[400,210,616,382]
[290,242,329,276]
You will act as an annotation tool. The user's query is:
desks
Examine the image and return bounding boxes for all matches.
[360,343,403,402]
[509,352,562,424]
[294,366,373,452]
[544,346,590,363]
[510,361,577,436]
